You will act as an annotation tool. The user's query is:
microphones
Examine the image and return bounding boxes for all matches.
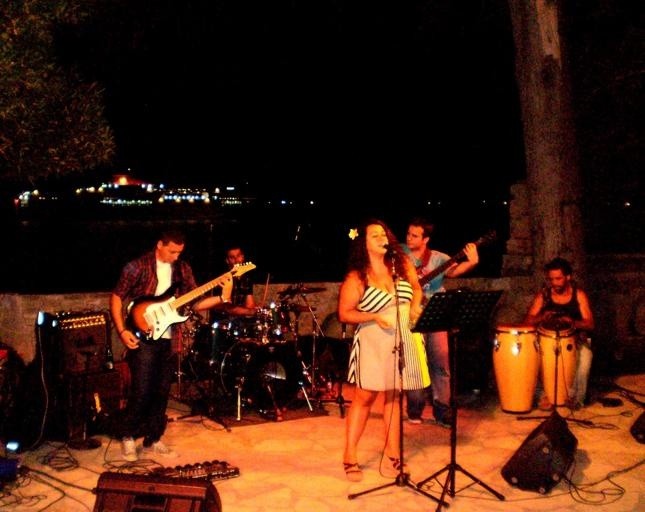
[382,242,397,254]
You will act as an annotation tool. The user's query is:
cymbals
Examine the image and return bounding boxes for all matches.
[281,287,326,293]
[279,303,317,312]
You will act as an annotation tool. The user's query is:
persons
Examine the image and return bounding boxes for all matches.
[400,220,479,429]
[208,245,256,322]
[523,257,596,412]
[108,228,233,462]
[337,217,424,483]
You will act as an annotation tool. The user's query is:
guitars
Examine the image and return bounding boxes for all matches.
[125,261,257,342]
[417,231,498,305]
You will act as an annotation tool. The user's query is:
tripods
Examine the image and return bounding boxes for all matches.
[349,273,449,507]
[321,324,352,418]
[416,328,504,512]
[515,330,595,425]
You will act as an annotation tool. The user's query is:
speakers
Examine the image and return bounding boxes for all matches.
[92,472,221,512]
[500,411,578,493]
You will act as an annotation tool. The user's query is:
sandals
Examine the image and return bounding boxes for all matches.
[343,461,362,482]
[389,456,407,471]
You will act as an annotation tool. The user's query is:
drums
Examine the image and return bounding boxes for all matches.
[190,322,235,358]
[492,325,540,413]
[256,305,289,333]
[219,335,303,407]
[538,320,577,404]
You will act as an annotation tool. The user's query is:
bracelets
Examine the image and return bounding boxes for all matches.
[571,318,576,329]
[412,281,421,291]
[118,328,126,335]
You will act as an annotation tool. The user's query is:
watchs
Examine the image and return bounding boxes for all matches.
[219,295,228,305]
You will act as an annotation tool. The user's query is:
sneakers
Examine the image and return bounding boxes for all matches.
[143,441,177,457]
[121,436,138,462]
